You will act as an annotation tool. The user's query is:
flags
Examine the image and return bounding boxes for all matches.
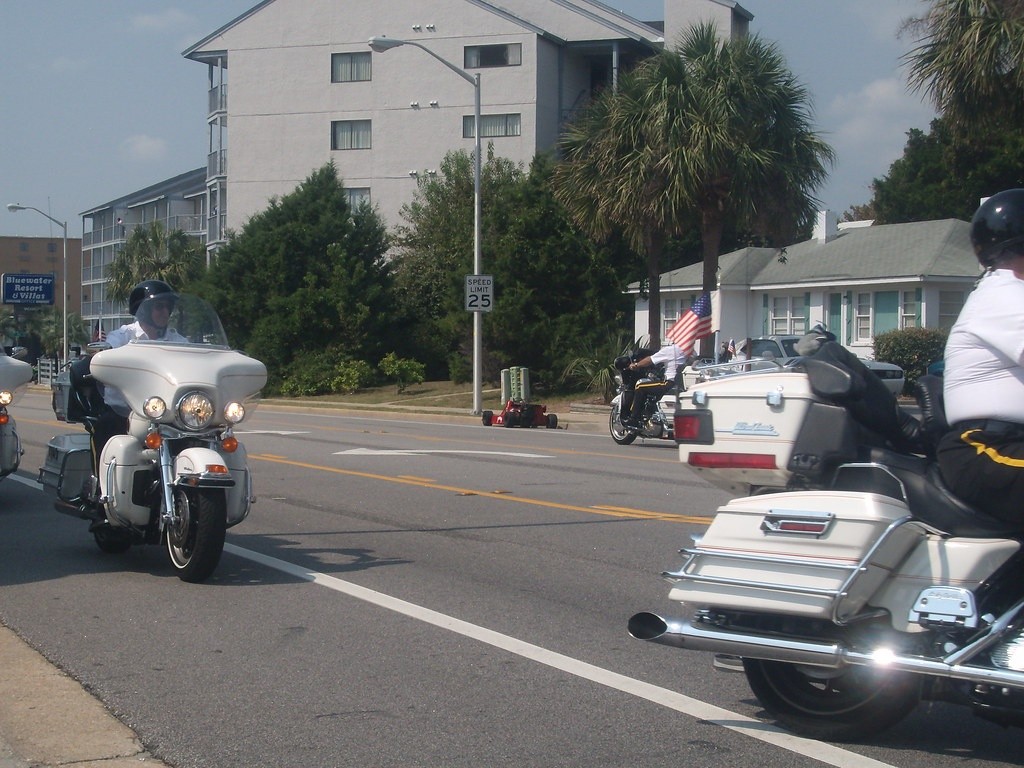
[727,339,737,357]
[665,293,712,357]
[92,320,105,351]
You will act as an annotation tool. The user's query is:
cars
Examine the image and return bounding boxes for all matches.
[731,336,802,367]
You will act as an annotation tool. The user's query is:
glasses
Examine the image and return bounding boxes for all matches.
[153,302,172,311]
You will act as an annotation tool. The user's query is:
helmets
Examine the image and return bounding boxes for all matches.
[129,281,178,316]
[970,188,1024,268]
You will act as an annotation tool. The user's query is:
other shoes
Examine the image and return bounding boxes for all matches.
[622,417,639,427]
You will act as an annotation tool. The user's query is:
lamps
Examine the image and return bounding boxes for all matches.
[411,100,438,106]
[412,24,434,29]
[409,170,437,175]
[641,296,648,302]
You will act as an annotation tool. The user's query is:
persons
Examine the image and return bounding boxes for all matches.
[81,281,193,498]
[941,190,1024,531]
[621,342,697,427]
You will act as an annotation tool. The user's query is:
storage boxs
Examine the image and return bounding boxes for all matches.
[43,434,94,499]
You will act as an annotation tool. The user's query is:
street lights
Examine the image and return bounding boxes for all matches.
[369,37,483,416]
[7,203,69,372]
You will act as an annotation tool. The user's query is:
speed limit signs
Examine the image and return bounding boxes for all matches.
[464,274,492,313]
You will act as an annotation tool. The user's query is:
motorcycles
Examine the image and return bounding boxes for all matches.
[36,292,269,584]
[0,344,33,483]
[608,333,712,446]
[623,329,1023,744]
